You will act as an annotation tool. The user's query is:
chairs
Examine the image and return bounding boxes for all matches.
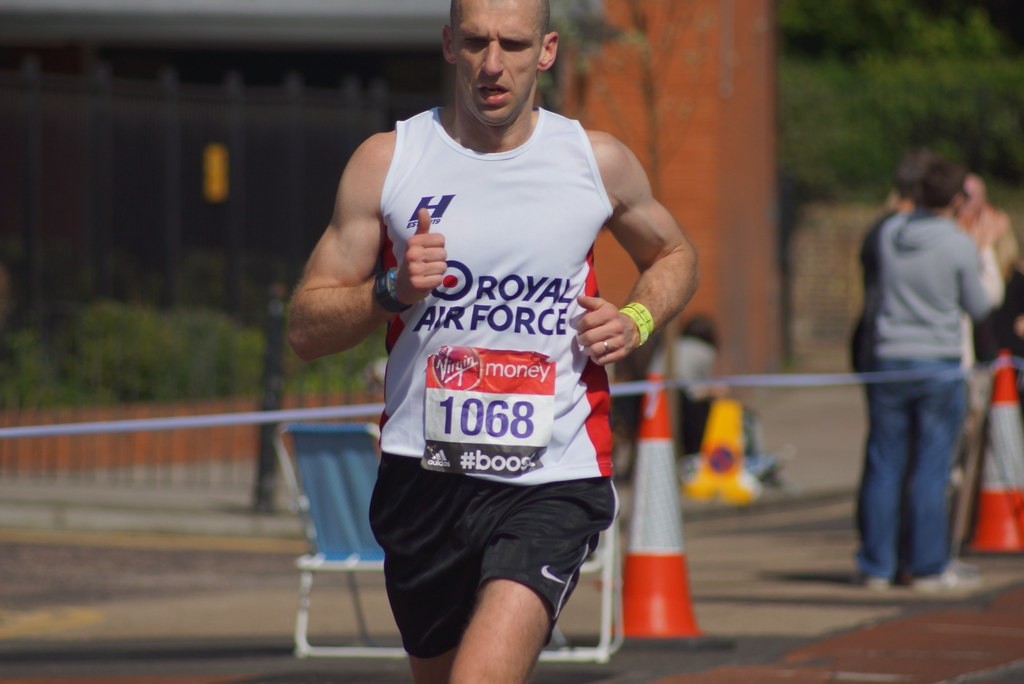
[271,421,627,663]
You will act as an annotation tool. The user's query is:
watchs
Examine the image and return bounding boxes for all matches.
[373,268,414,312]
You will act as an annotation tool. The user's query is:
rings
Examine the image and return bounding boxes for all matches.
[603,341,610,353]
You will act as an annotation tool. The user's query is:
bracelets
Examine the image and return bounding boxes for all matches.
[619,302,655,348]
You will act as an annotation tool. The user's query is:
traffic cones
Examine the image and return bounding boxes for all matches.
[681,387,778,511]
[616,372,745,653]
[961,348,1024,565]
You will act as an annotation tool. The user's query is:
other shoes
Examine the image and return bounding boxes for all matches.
[865,573,889,593]
[902,566,958,590]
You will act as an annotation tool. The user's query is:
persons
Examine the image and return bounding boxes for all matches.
[668,315,785,489]
[849,148,1024,592]
[288,0,699,684]
